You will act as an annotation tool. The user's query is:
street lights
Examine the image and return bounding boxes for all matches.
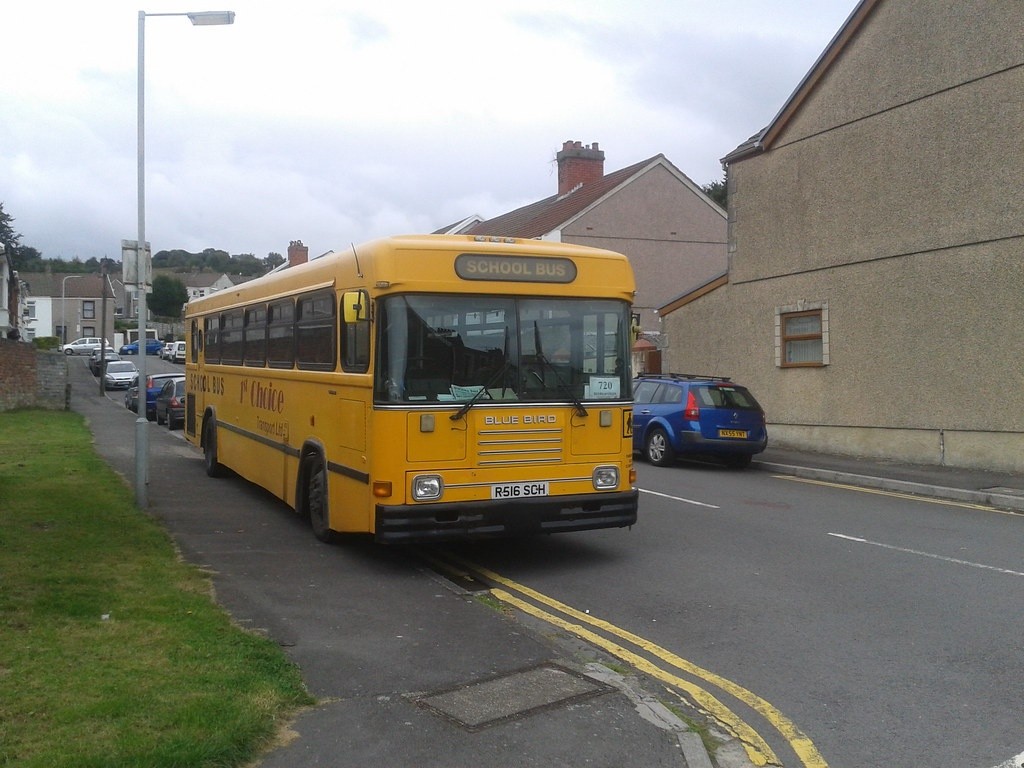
[135,8,239,509]
[61,275,85,344]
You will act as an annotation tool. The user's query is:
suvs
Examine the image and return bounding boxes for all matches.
[632,372,770,468]
[145,373,185,422]
[57,337,110,356]
[168,341,186,363]
[89,347,115,369]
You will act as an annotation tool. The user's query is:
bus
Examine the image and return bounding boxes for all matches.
[181,232,640,555]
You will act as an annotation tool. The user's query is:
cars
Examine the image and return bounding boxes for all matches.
[159,342,174,360]
[156,377,186,431]
[91,353,123,377]
[105,360,139,391]
[124,374,139,413]
[120,337,166,356]
